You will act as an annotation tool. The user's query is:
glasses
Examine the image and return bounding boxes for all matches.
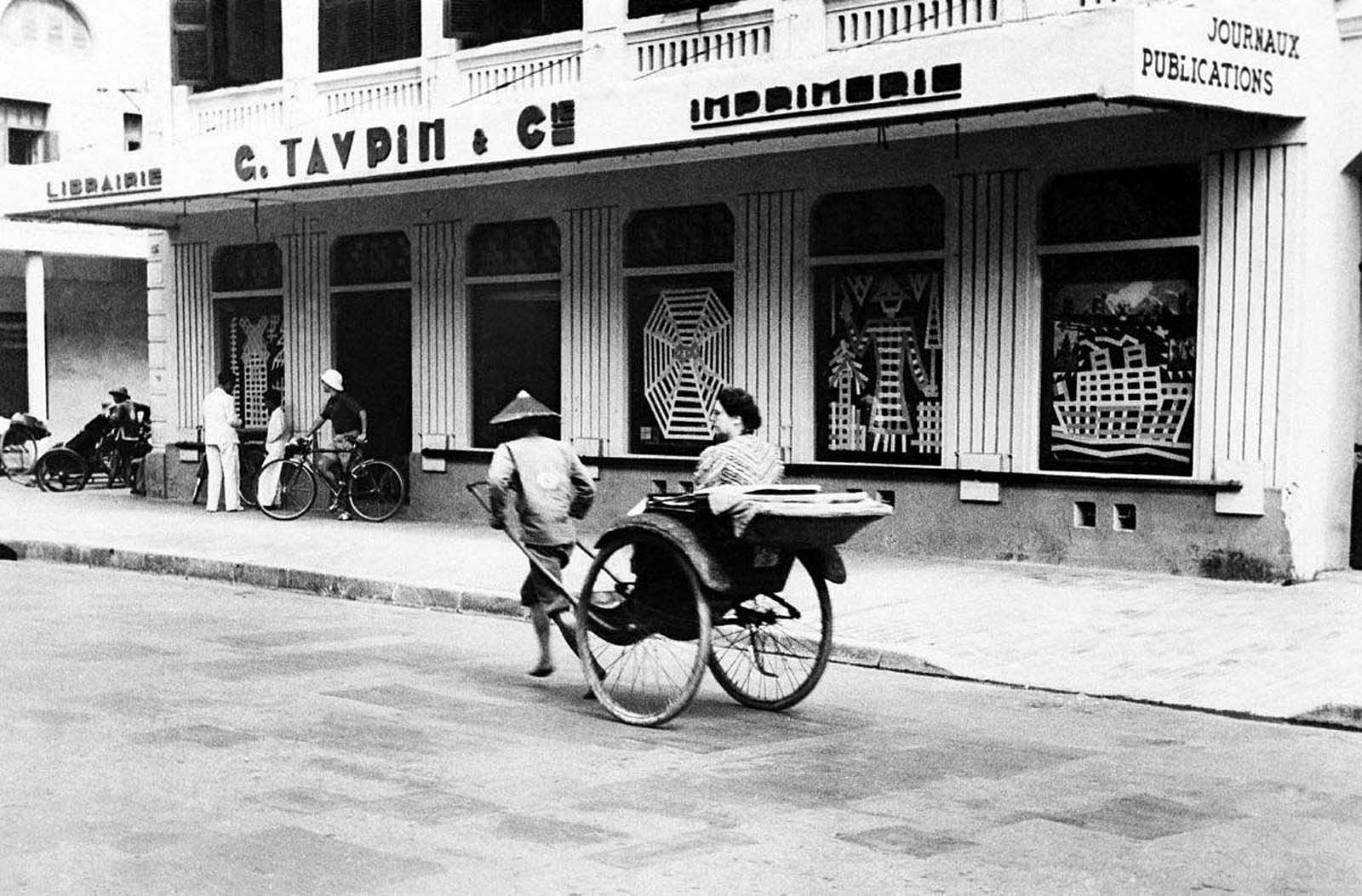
[262,399,269,402]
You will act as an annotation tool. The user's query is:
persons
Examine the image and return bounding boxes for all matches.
[255,390,289,508]
[296,368,367,522]
[612,388,783,609]
[105,387,150,485]
[198,369,245,513]
[485,390,609,701]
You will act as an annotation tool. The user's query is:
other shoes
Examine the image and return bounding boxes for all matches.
[583,674,605,699]
[527,668,553,677]
[328,502,340,510]
[338,511,352,521]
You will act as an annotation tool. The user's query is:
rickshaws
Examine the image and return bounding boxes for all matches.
[0,413,50,477]
[467,479,895,724]
[35,402,151,493]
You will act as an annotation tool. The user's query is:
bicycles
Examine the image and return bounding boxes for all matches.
[253,438,406,522]
[192,439,266,507]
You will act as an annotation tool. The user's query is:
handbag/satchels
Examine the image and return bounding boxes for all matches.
[197,454,207,479]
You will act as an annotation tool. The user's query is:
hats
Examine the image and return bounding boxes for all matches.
[489,390,562,426]
[320,368,344,391]
[262,387,281,404]
[109,387,131,400]
